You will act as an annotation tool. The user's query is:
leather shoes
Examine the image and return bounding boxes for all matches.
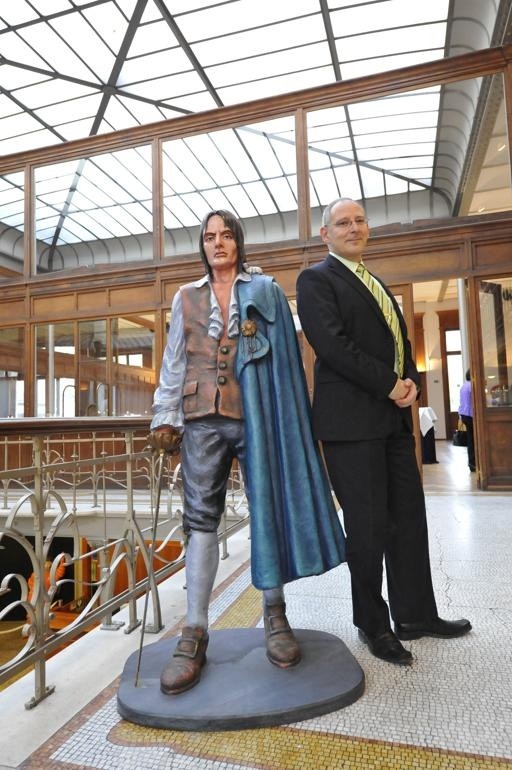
[358,627,412,666]
[396,619,471,641]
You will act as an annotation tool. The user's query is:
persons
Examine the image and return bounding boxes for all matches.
[148,209,346,696]
[457,367,478,472]
[295,195,475,665]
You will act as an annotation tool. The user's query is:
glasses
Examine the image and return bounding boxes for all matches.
[328,216,370,227]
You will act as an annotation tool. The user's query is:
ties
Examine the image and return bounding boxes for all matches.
[356,266,405,379]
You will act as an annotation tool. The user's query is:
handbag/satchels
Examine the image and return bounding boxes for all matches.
[452,430,468,446]
